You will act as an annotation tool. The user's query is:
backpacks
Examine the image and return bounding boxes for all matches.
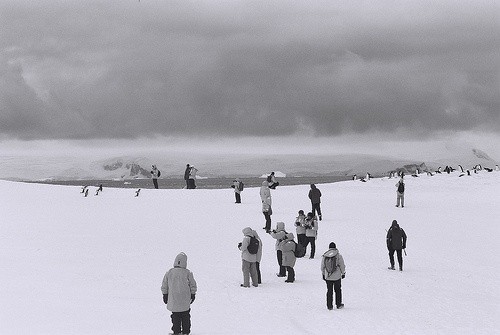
[246,235,259,254]
[398,182,404,193]
[322,255,337,277]
[158,170,161,178]
[267,175,272,182]
[289,241,306,258]
[237,181,244,191]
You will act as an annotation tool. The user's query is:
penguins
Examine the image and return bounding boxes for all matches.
[495,164,500,170]
[423,170,434,176]
[444,165,456,174]
[471,164,494,174]
[387,170,405,179]
[411,174,419,178]
[434,166,442,175]
[352,171,375,183]
[466,168,471,176]
[457,164,464,173]
[134,188,142,197]
[79,183,103,198]
[415,168,420,174]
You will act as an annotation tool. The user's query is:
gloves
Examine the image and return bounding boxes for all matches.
[402,245,406,249]
[238,245,241,248]
[238,242,242,245]
[162,294,168,305]
[190,294,196,304]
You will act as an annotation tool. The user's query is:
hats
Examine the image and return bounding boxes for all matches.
[187,164,190,166]
[392,220,397,226]
[329,241,336,248]
[272,172,274,174]
[307,212,313,216]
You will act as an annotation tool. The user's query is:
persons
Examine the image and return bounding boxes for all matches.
[320,242,346,310]
[161,251,198,335]
[184,164,199,189]
[268,172,280,189]
[234,178,241,203]
[239,227,258,287]
[294,209,307,243]
[386,219,407,271]
[252,230,262,284]
[270,222,287,277]
[309,183,322,221]
[303,213,318,259]
[278,232,296,283]
[395,178,405,208]
[260,181,272,230]
[151,164,158,188]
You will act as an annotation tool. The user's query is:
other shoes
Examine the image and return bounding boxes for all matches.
[277,274,285,277]
[402,205,404,207]
[285,280,293,283]
[241,284,250,288]
[252,284,258,287]
[337,304,344,309]
[388,266,395,270]
[329,306,333,310]
[396,205,398,207]
